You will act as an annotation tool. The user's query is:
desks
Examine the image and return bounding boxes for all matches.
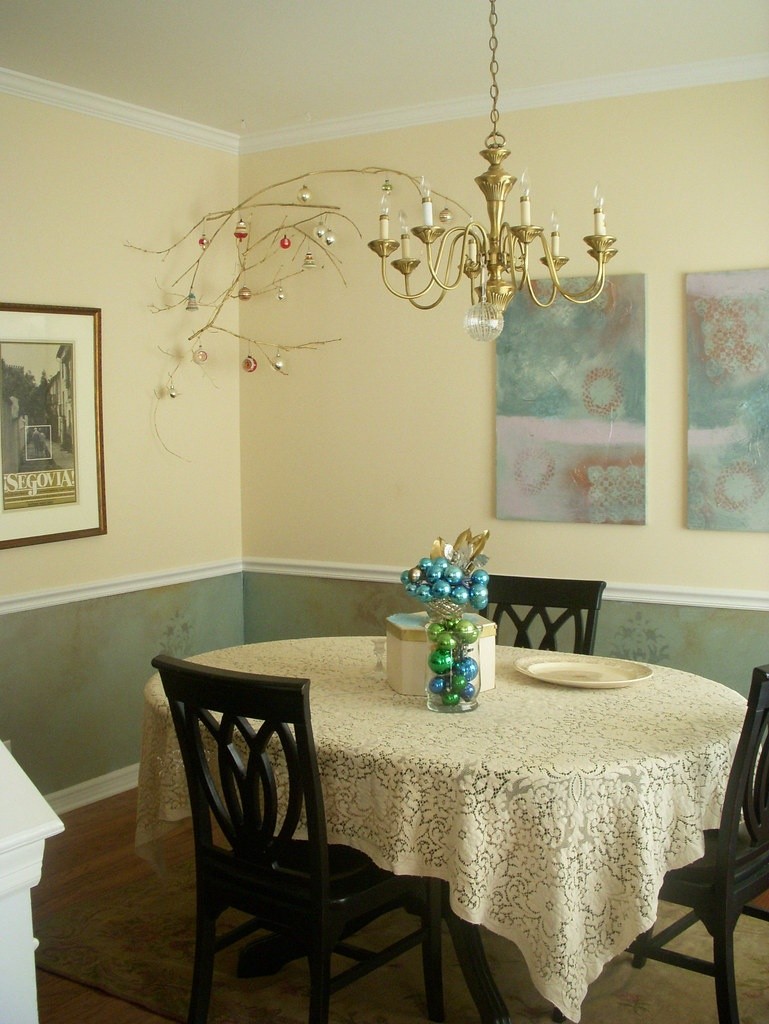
[129,634,763,1024]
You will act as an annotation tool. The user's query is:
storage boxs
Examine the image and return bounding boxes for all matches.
[386,611,496,696]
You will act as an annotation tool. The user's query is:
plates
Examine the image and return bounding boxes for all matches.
[512,654,653,690]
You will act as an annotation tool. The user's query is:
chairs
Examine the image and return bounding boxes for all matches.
[149,652,447,1024]
[552,660,769,1024]
[481,575,606,655]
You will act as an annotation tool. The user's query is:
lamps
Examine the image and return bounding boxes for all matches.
[368,0,619,341]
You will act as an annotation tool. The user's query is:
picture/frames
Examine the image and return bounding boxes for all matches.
[0,303,108,550]
